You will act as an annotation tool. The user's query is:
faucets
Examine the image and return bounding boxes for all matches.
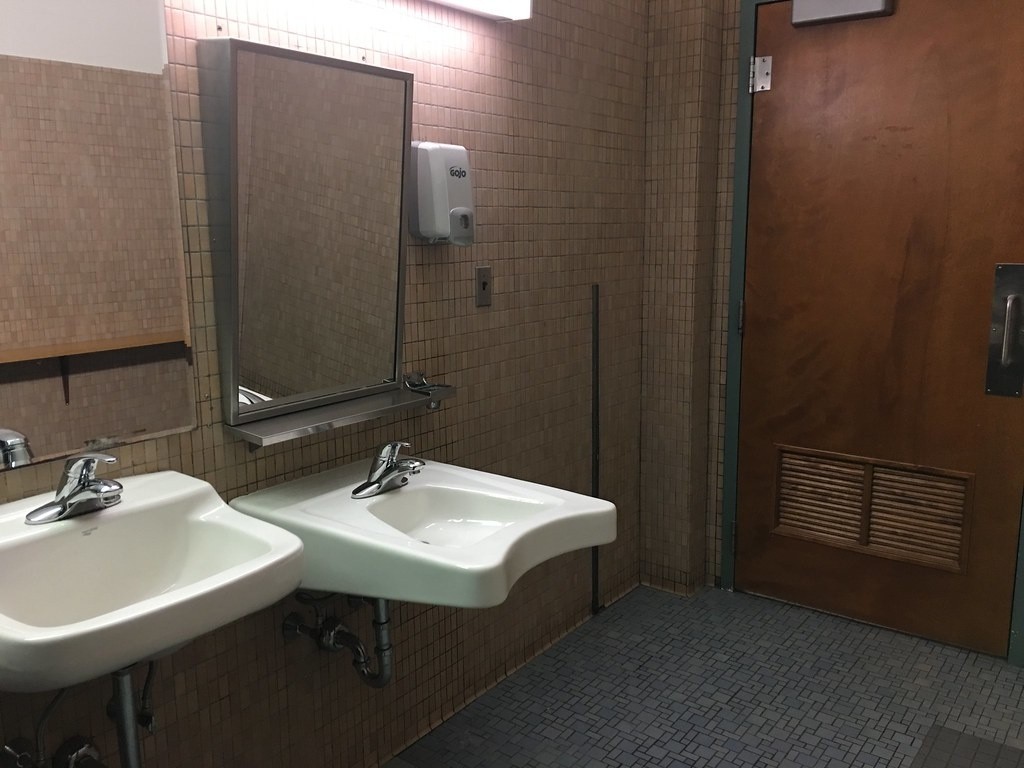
[238,386,274,409]
[351,441,427,500]
[0,427,34,468]
[25,451,123,525]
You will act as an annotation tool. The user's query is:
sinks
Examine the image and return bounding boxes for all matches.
[0,471,303,694]
[229,455,617,609]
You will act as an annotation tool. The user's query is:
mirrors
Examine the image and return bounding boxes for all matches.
[197,35,415,429]
[0,0,200,476]
